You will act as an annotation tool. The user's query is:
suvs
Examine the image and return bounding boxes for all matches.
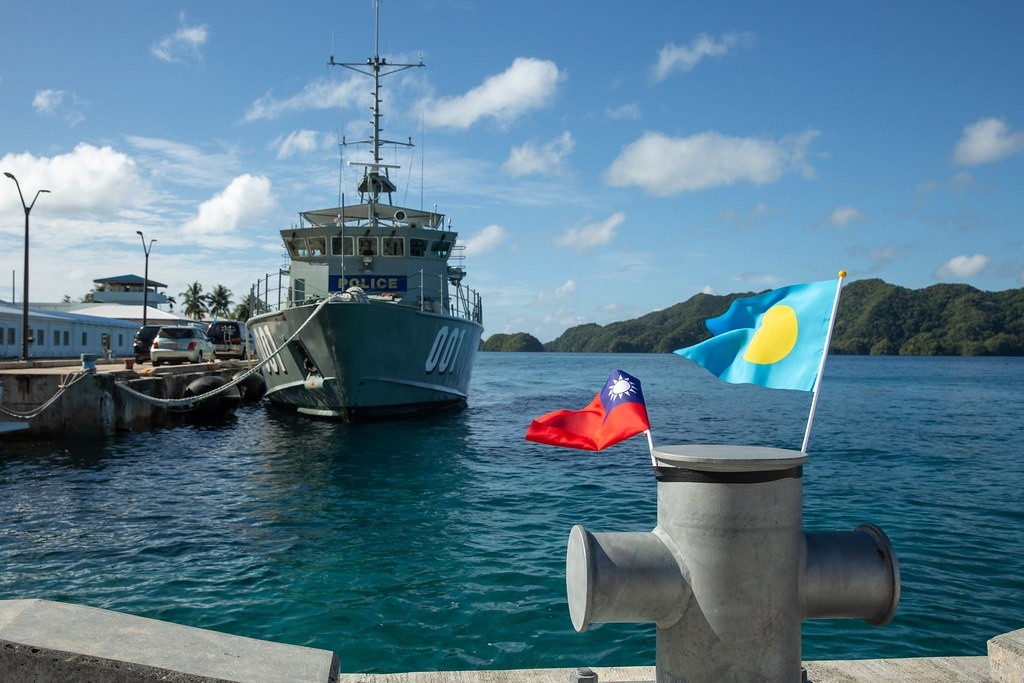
[133,325,180,364]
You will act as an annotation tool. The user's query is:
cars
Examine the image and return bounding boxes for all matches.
[150,326,216,367]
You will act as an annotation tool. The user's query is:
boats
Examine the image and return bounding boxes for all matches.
[243,1,484,421]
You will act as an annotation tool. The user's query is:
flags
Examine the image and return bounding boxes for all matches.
[672,279,842,393]
[523,368,653,453]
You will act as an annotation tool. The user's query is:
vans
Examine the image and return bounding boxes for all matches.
[206,321,256,360]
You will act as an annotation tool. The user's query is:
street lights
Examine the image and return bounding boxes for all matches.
[136,231,158,326]
[3,172,51,360]
